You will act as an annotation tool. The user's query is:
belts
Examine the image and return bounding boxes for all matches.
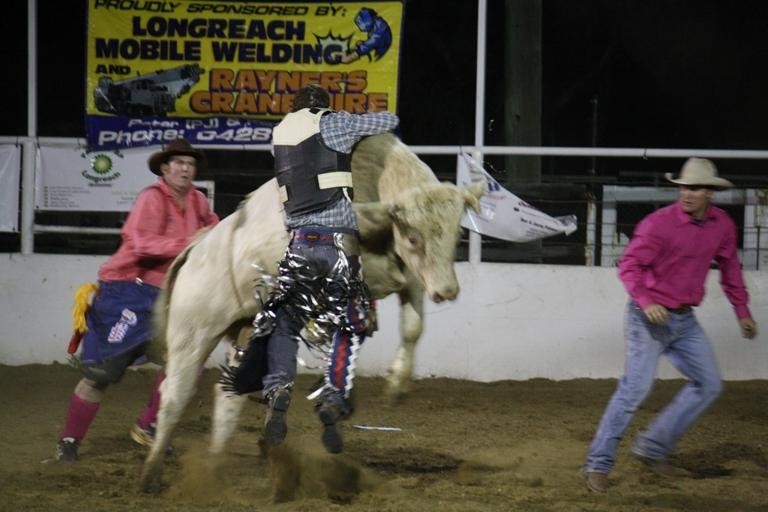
[666,306,693,314]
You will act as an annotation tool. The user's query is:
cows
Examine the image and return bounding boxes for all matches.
[138,130,491,495]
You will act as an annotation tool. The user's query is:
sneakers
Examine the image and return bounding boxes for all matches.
[54,436,77,461]
[638,455,673,476]
[264,386,290,446]
[319,403,344,454]
[584,472,610,494]
[131,424,172,452]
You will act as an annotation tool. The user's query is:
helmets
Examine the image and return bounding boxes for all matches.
[291,83,329,111]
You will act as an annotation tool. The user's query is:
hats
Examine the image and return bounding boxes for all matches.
[665,156,734,191]
[149,139,203,176]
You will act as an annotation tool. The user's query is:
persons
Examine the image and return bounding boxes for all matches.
[56,138,220,460]
[583,158,757,495]
[246,84,399,453]
